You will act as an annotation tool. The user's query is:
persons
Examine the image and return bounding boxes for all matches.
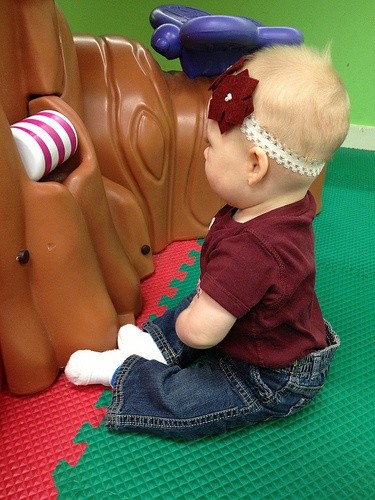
[65,41,352,441]
